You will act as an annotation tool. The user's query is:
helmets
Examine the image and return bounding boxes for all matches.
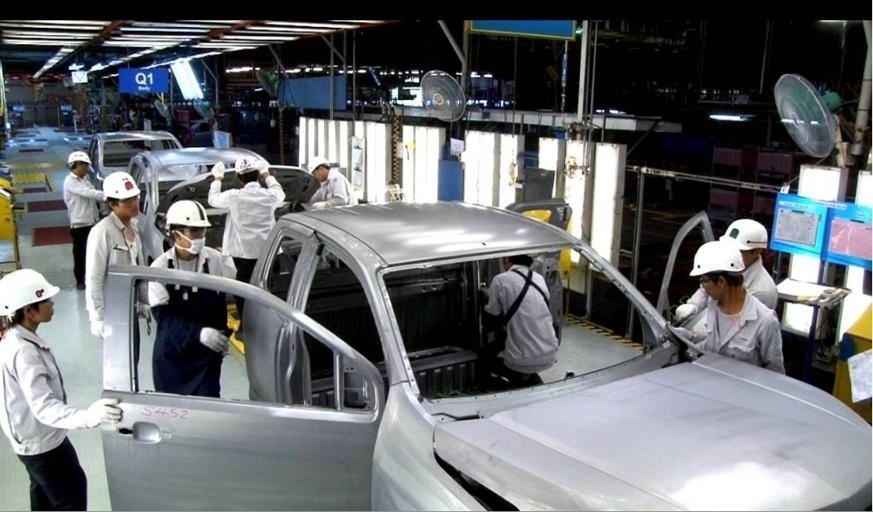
[689,241,745,277]
[68,151,92,167]
[235,157,257,175]
[719,219,768,250]
[103,172,141,202]
[309,158,327,174]
[0,269,60,321]
[166,200,211,235]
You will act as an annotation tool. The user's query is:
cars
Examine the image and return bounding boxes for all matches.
[90,130,182,215]
[103,197,871,512]
[129,146,319,265]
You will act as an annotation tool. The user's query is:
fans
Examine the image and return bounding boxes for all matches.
[254,68,277,95]
[776,72,848,172]
[420,70,468,124]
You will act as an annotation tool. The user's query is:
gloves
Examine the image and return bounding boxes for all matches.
[256,160,269,173]
[91,321,104,335]
[86,398,122,428]
[312,201,330,209]
[674,326,692,339]
[200,327,228,353]
[212,162,225,178]
[675,304,697,319]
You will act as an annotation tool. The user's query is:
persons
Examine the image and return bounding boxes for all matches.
[0,267,125,511]
[59,149,106,292]
[682,239,787,377]
[301,156,360,211]
[84,171,149,365]
[482,255,560,376]
[147,198,237,400]
[207,155,287,332]
[675,217,779,343]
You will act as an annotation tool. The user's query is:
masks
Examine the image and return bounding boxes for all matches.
[171,230,205,255]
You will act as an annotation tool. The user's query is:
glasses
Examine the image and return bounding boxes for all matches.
[701,276,712,288]
